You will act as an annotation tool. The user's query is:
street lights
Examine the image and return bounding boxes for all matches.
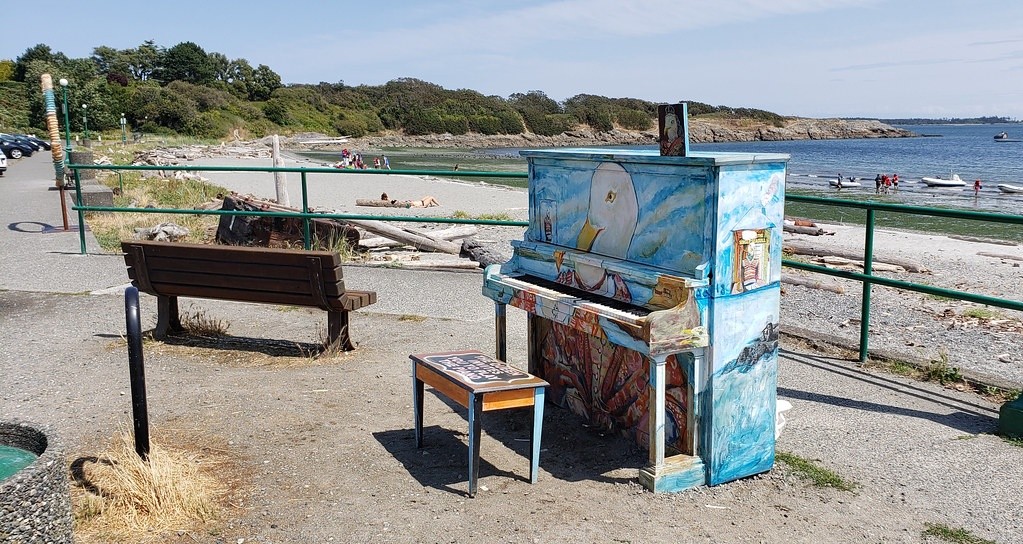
[59,79,72,163]
[120,113,126,145]
[81,103,89,139]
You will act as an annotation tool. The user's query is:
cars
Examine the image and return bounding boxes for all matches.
[0,133,52,175]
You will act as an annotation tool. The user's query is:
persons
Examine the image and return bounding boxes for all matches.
[382,155,391,170]
[374,157,381,169]
[455,164,458,170]
[973,180,979,195]
[837,174,841,188]
[893,174,899,190]
[406,196,440,208]
[850,178,855,182]
[875,174,891,194]
[344,153,362,169]
[381,193,388,200]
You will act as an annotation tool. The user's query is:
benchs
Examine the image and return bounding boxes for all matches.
[120,238,378,354]
[409,349,550,500]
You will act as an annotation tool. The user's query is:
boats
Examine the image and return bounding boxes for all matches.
[997,183,1023,194]
[829,179,862,188]
[919,169,967,187]
[993,131,1023,142]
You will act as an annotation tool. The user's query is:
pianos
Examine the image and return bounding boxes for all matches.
[480,145,792,496]
